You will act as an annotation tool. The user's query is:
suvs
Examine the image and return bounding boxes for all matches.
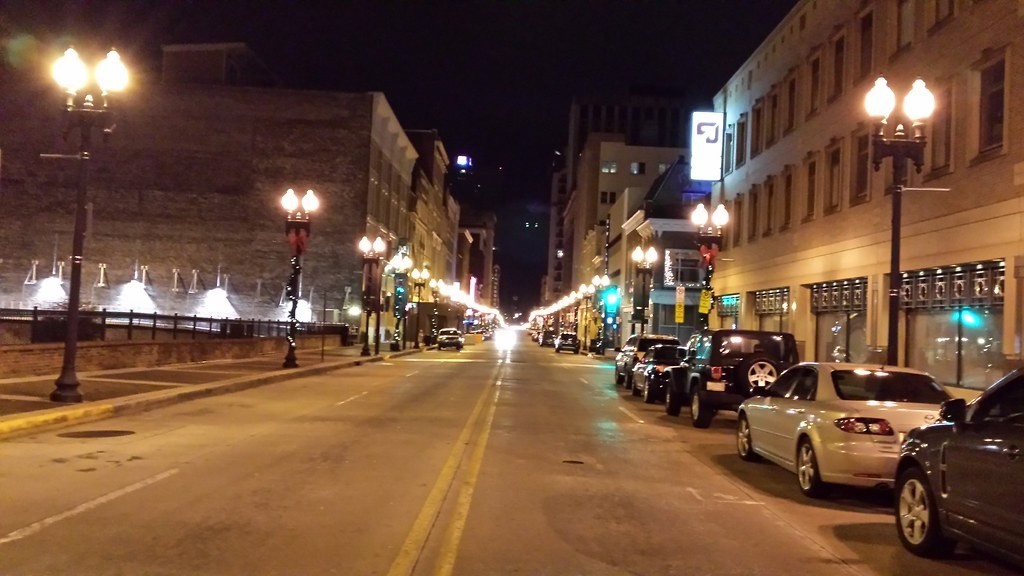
[662,330,800,429]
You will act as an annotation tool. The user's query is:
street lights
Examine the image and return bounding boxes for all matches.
[281,188,319,368]
[692,204,729,329]
[632,246,658,333]
[570,274,610,355]
[359,236,385,357]
[50,49,127,403]
[392,256,412,350]
[429,279,444,346]
[863,72,935,367]
[412,268,429,349]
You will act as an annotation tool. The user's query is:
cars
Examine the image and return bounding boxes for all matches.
[737,362,961,498]
[437,328,465,350]
[894,366,1024,573]
[538,331,556,346]
[554,332,580,354]
[532,332,540,342]
[613,336,683,390]
[631,345,697,403]
